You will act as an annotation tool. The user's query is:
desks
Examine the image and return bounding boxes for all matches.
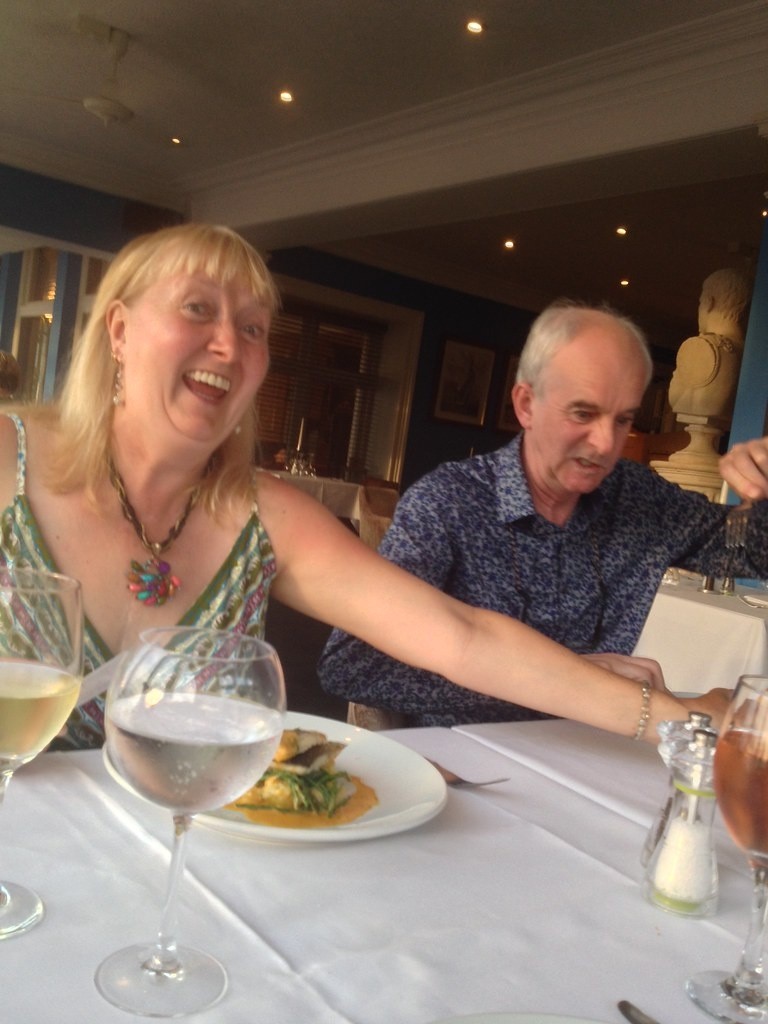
[631,573,768,692]
[257,468,401,519]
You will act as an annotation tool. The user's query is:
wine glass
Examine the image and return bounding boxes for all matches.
[682,674,767,1023]
[94,626,286,1019]
[301,452,317,479]
[0,564,85,938]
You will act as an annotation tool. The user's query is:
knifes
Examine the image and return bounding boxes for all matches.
[618,1001,661,1024]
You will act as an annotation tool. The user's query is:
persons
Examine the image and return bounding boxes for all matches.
[260,443,290,471]
[319,300,768,729]
[0,351,19,402]
[668,268,749,418]
[0,223,733,756]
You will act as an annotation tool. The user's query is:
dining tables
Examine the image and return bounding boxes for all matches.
[0,717,768,1024]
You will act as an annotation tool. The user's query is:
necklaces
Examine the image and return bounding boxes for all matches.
[106,459,215,608]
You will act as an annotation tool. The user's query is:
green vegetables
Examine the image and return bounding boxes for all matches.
[233,764,355,817]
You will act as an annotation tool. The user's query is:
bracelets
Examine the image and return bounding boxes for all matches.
[635,681,651,739]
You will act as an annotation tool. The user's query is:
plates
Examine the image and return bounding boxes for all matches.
[101,710,448,843]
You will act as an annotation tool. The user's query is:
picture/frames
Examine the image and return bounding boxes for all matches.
[433,339,495,426]
[497,356,528,432]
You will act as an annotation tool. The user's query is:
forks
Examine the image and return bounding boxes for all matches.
[424,755,510,790]
[726,499,752,548]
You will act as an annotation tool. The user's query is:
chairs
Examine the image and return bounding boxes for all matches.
[357,486,398,548]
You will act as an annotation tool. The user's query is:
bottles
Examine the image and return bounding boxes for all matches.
[644,729,722,917]
[641,710,715,865]
